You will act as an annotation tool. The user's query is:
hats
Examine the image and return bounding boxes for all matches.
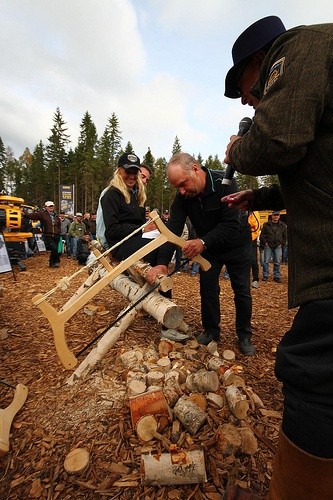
[272,211,280,215]
[118,152,142,173]
[224,16,286,99]
[76,213,82,216]
[44,200,55,207]
[163,210,169,214]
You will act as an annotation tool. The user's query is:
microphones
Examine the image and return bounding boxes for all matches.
[221,116,252,188]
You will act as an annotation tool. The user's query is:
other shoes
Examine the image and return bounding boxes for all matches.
[55,263,59,267]
[224,272,229,279]
[274,277,282,282]
[67,256,72,259]
[262,276,268,280]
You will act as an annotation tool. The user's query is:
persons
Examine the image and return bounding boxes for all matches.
[59,210,73,259]
[218,14,333,500]
[77,231,93,265]
[246,210,261,288]
[89,211,96,237]
[24,200,61,269]
[101,153,189,341]
[69,212,87,260]
[95,164,161,251]
[83,212,92,230]
[166,152,255,356]
[259,209,288,283]
[151,207,201,276]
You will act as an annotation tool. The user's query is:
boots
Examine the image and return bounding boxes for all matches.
[267,422,333,500]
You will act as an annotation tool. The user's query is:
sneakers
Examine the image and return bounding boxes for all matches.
[238,338,254,355]
[196,330,219,345]
[252,281,258,287]
[161,328,188,341]
